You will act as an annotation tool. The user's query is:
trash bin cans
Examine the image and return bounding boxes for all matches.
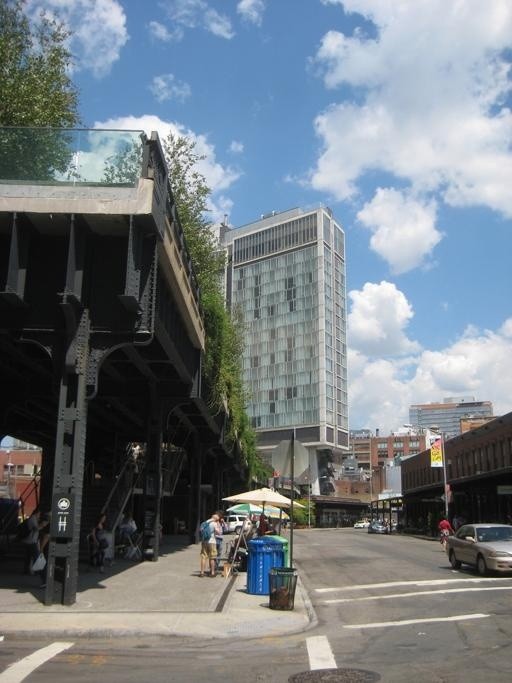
[247,535,288,595]
[269,567,298,610]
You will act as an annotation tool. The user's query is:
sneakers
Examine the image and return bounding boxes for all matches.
[199,568,221,578]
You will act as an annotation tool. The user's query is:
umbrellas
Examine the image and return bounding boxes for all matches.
[225,503,263,518]
[257,505,290,523]
[222,486,307,535]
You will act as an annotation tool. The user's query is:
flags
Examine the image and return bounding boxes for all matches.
[430,435,443,469]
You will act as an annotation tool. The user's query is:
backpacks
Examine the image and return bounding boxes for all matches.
[16,518,35,539]
[200,520,216,543]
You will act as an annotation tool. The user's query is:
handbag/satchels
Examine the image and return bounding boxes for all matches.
[96,536,110,550]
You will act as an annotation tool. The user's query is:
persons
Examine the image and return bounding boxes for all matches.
[426,510,466,550]
[39,513,52,588]
[93,512,109,573]
[119,513,140,561]
[214,511,228,570]
[197,513,221,577]
[257,513,278,534]
[22,507,50,575]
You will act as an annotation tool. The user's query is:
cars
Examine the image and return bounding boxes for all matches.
[446,522,512,573]
[354,517,398,535]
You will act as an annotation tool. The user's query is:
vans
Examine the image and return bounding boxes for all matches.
[222,512,252,534]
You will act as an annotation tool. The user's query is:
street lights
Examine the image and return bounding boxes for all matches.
[6,449,13,498]
[402,424,449,523]
